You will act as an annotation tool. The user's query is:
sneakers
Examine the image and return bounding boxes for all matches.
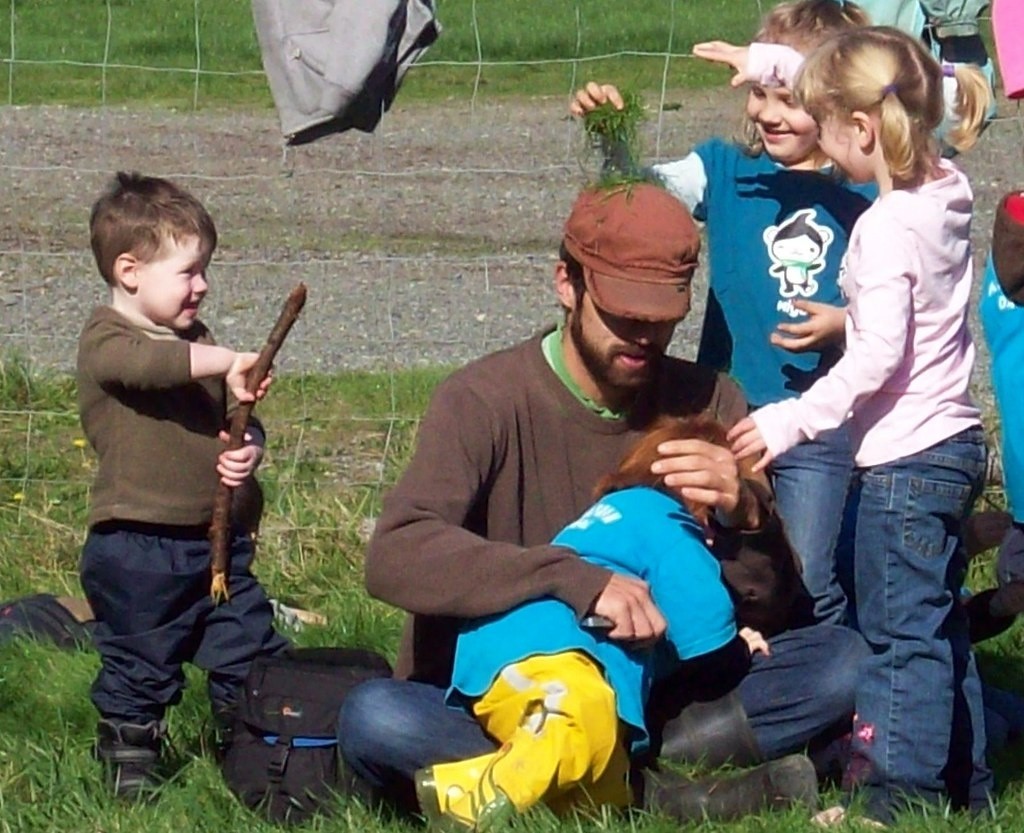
[91,716,164,800]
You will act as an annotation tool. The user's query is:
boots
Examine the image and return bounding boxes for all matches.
[642,693,765,771]
[638,754,819,828]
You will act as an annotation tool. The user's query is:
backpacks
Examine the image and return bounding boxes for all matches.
[212,644,394,830]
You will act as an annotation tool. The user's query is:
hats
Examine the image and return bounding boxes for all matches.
[565,177,701,321]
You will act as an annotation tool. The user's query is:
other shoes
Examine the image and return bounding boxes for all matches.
[805,802,888,831]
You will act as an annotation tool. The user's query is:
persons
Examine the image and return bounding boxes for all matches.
[338,0,1024,833]
[76,170,292,799]
